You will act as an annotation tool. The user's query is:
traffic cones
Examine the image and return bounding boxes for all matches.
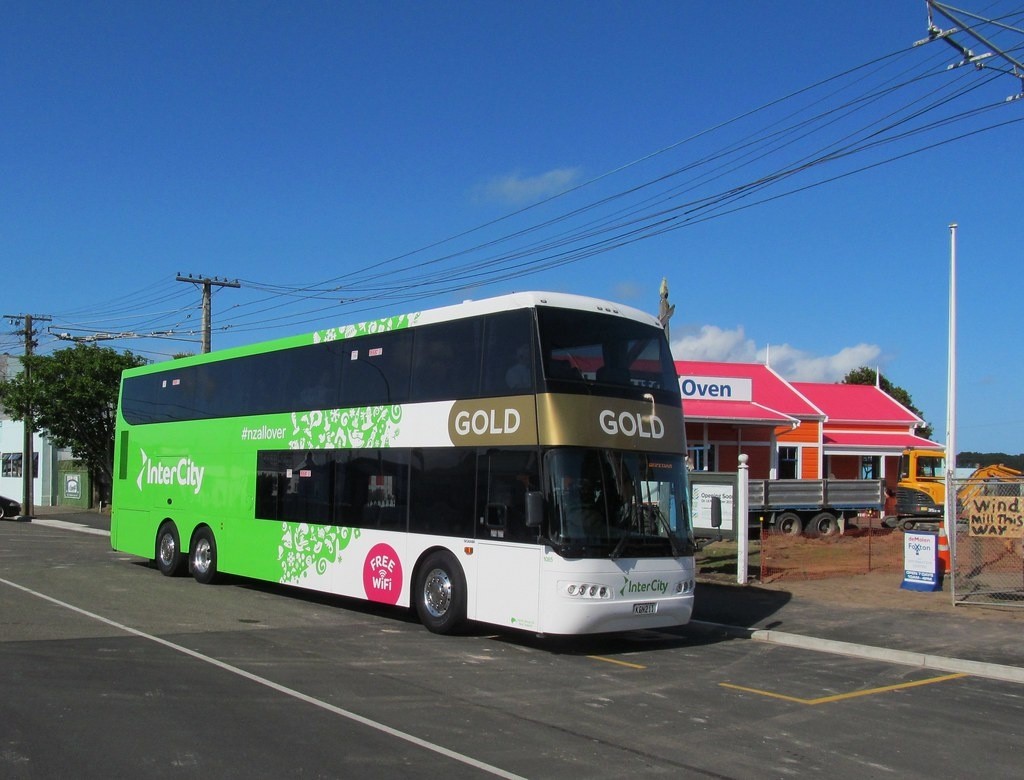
[938,520,951,578]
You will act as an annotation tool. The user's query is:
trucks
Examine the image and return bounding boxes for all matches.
[749,478,885,541]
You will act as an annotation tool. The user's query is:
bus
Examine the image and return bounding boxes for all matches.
[111,290,723,637]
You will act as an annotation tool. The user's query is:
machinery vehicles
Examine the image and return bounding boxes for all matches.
[881,446,1024,532]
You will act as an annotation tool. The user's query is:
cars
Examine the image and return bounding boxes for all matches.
[0,495,21,520]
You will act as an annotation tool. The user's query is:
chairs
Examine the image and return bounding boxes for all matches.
[595,365,633,395]
